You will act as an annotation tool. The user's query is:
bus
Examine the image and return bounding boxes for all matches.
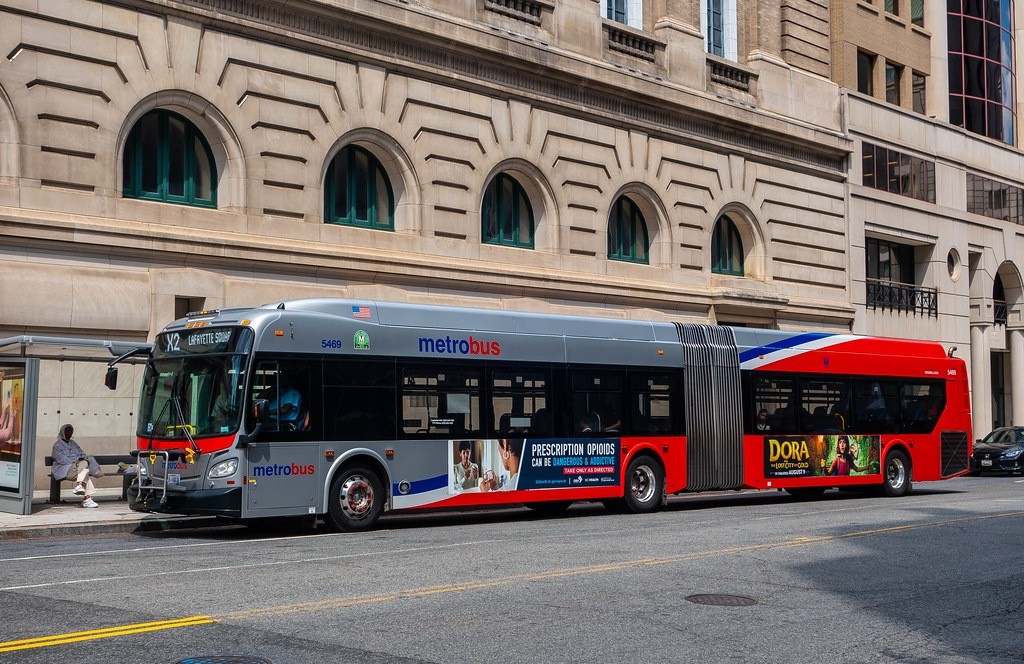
[105,300,973,529]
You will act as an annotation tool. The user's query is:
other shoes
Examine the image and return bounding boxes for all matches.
[72,485,85,495]
[82,497,98,508]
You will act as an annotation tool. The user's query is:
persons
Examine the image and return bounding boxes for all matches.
[756,409,770,430]
[577,393,621,432]
[51,424,99,508]
[8,383,22,441]
[454,439,524,492]
[261,361,302,420]
[0,401,13,447]
[821,436,870,475]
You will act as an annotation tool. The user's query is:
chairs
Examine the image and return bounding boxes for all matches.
[296,369,311,431]
[586,410,601,432]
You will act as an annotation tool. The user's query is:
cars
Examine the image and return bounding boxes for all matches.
[972,426,1024,476]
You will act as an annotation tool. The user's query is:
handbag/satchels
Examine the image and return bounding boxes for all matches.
[83,455,103,478]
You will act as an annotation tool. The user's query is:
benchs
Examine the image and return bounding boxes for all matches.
[812,413,845,433]
[499,413,536,432]
[44,454,137,505]
